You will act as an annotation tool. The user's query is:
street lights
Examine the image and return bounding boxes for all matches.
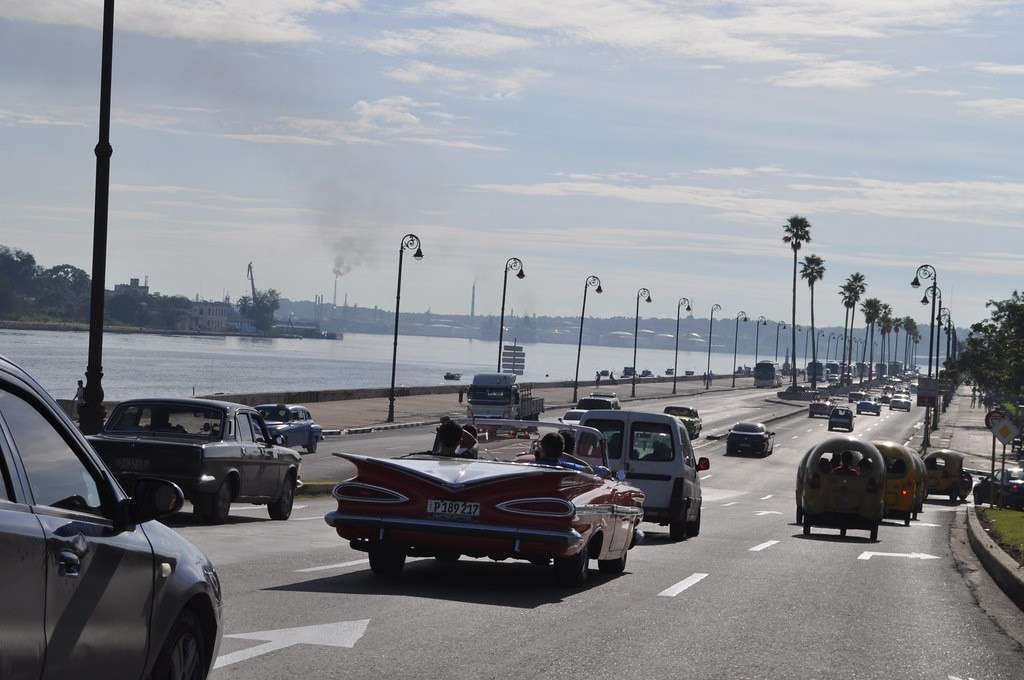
[774,320,787,360]
[572,274,602,402]
[732,311,748,388]
[387,233,424,420]
[632,288,652,399]
[492,257,526,374]
[837,334,877,363]
[920,286,943,431]
[912,264,937,444]
[755,315,767,365]
[706,303,721,389]
[673,297,691,395]
[816,329,825,361]
[792,324,801,381]
[826,331,836,361]
[936,308,952,414]
[943,321,959,403]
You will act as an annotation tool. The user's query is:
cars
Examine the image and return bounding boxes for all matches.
[863,439,923,527]
[916,449,971,506]
[726,421,775,458]
[889,393,912,412]
[559,410,589,428]
[0,352,224,679]
[829,374,838,383]
[73,397,304,516]
[971,468,1024,509]
[661,403,700,437]
[250,403,325,454]
[880,370,928,403]
[827,407,856,430]
[797,439,889,540]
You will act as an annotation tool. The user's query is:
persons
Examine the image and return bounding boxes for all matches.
[964,378,994,410]
[927,457,938,469]
[818,457,833,474]
[610,372,615,385]
[162,413,176,431]
[529,433,595,474]
[558,428,611,478]
[73,380,84,424]
[634,370,641,384]
[260,409,301,422]
[594,371,600,390]
[508,428,517,439]
[410,415,480,459]
[702,372,708,386]
[580,400,612,409]
[816,396,836,404]
[884,383,896,396]
[867,398,871,401]
[898,387,909,390]
[708,370,713,386]
[874,398,878,402]
[830,452,842,469]
[861,397,865,401]
[454,423,478,458]
[856,454,873,475]
[503,387,511,399]
[686,411,693,416]
[831,451,859,476]
[853,388,867,393]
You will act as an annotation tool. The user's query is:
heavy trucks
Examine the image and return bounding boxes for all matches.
[917,376,938,407]
[463,373,545,427]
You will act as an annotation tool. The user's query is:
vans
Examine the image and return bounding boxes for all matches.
[567,408,702,536]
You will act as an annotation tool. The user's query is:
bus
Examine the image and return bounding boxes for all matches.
[825,361,840,381]
[807,361,823,382]
[753,360,782,388]
[839,361,905,377]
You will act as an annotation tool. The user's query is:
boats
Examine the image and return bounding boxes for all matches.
[443,372,462,380]
[733,363,754,376]
[600,370,610,376]
[639,369,654,377]
[622,367,638,378]
[665,368,674,376]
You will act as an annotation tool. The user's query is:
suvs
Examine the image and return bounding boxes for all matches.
[838,373,853,383]
[578,390,620,412]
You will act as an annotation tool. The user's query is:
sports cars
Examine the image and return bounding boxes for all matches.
[808,398,834,416]
[856,398,882,415]
[849,388,869,402]
[326,417,649,582]
